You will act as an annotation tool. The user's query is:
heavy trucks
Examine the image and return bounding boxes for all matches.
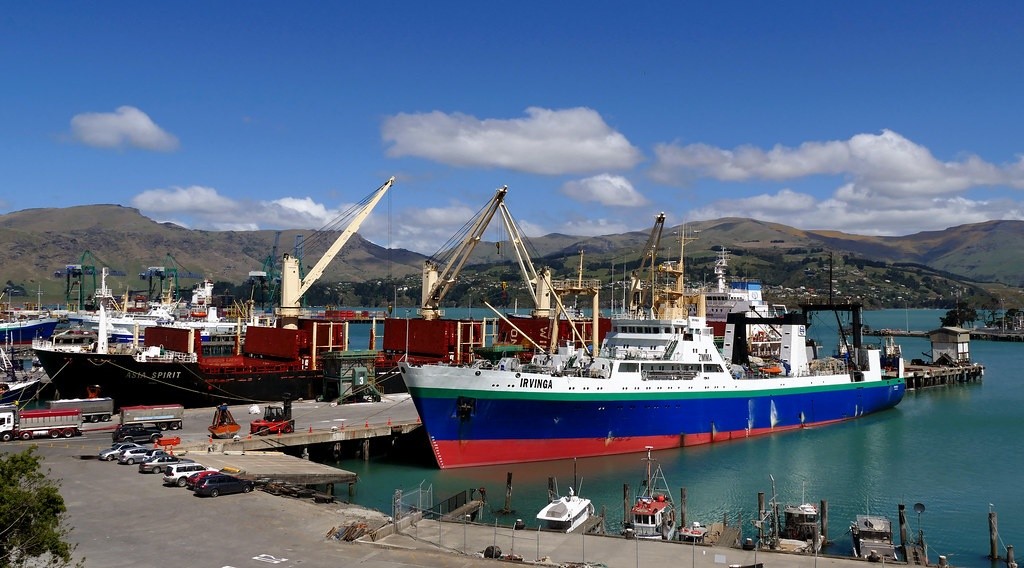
[0,407,83,441]
[30,397,114,422]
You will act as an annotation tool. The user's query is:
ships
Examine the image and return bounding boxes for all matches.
[29,242,812,410]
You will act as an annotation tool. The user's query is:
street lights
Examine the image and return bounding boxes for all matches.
[913,503,926,545]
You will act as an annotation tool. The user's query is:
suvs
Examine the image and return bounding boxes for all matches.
[164,463,219,487]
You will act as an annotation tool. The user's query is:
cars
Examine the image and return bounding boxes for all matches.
[98,442,147,462]
[139,455,196,473]
[910,359,925,365]
[187,471,220,489]
[118,447,151,465]
[142,449,170,462]
[196,475,255,498]
[112,424,164,445]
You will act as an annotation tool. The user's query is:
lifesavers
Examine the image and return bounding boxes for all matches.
[691,531,700,534]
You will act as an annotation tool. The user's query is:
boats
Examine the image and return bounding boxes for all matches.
[48,267,262,350]
[848,513,900,561]
[675,522,723,546]
[0,331,41,406]
[0,306,60,347]
[395,219,907,468]
[621,446,676,541]
[533,458,603,534]
[759,366,782,374]
[753,479,824,555]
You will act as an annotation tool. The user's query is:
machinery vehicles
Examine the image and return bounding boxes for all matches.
[250,392,295,434]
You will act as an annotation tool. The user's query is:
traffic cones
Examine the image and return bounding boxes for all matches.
[340,421,345,430]
[247,431,251,439]
[365,418,369,428]
[417,415,420,423]
[172,438,176,446]
[170,446,175,457]
[209,435,213,443]
[153,442,158,448]
[277,428,282,436]
[308,424,313,432]
[387,417,392,425]
